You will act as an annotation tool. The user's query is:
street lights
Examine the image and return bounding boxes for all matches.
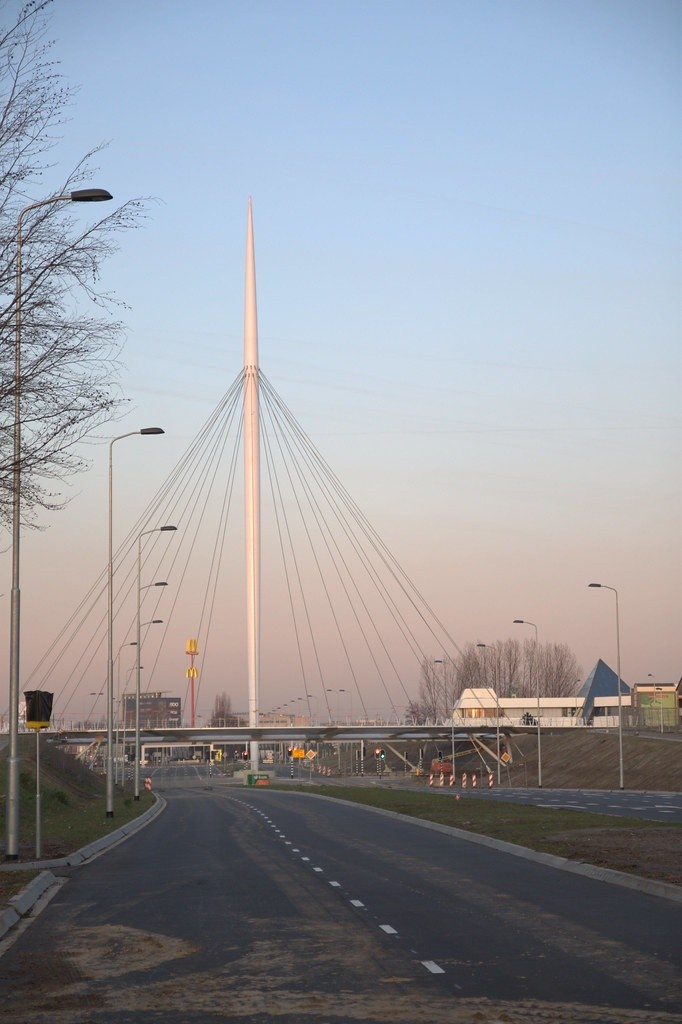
[648,674,655,707]
[436,659,456,783]
[513,620,543,787]
[115,643,138,786]
[5,189,115,860]
[477,644,501,785]
[588,583,623,789]
[134,526,178,798]
[123,666,142,787]
[107,428,167,818]
[656,687,663,733]
[340,690,352,725]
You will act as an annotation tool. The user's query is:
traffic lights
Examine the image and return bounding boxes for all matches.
[288,747,292,757]
[381,750,385,759]
[375,749,379,758]
[244,752,247,761]
[210,751,215,760]
[24,691,39,730]
[438,751,442,760]
